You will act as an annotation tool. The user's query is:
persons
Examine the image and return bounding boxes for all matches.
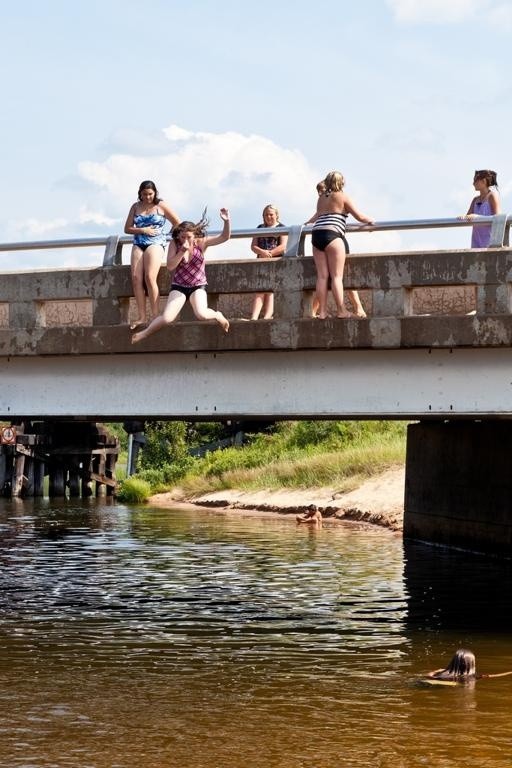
[131,206,231,345]
[457,170,502,317]
[303,170,375,320]
[415,649,512,689]
[296,504,323,524]
[250,204,288,321]
[124,180,182,330]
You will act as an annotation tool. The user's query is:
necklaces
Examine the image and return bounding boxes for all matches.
[477,190,491,205]
